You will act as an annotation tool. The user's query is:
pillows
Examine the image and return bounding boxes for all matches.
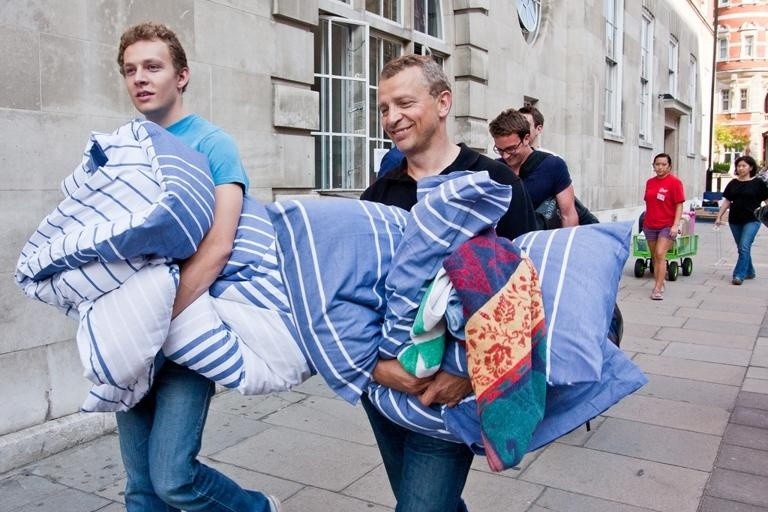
[502,218,637,389]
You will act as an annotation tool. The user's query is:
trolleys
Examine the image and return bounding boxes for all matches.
[634,234,703,281]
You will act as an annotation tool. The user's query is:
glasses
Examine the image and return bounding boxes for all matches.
[493,141,521,153]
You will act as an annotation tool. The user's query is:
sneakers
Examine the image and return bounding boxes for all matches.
[732,274,755,284]
[652,290,663,300]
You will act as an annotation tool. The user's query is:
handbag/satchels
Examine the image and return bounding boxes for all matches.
[534,195,599,229]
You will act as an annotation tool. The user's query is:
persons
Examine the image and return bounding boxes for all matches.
[643,153,684,299]
[490,109,580,227]
[112,22,279,512]
[355,51,537,511]
[714,155,767,286]
[519,102,546,149]
